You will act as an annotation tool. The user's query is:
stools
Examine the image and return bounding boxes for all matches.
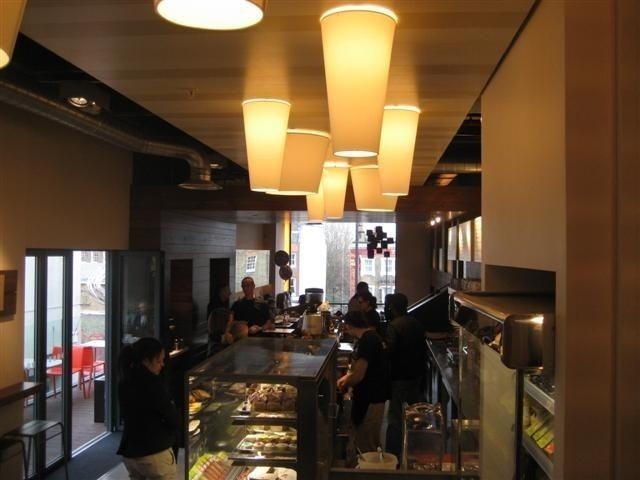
[3,420,69,480]
[0,439,27,480]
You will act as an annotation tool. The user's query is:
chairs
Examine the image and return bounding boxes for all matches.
[23,340,106,406]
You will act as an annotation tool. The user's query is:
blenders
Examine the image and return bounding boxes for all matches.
[301,288,331,338]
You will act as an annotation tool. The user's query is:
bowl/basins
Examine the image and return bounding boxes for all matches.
[358,452,398,470]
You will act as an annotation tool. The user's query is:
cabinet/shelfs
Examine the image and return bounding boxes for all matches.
[184,337,340,479]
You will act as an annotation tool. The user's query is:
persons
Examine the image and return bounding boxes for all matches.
[384,293,428,453]
[132,302,149,330]
[231,277,270,334]
[206,308,235,358]
[337,310,390,451]
[206,281,232,321]
[117,337,209,480]
[358,293,377,316]
[349,282,372,313]
[367,310,381,332]
[264,290,289,312]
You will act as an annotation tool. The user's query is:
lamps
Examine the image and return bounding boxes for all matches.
[318,10,398,158]
[242,100,291,190]
[351,166,398,212]
[264,130,330,196]
[154,1,265,31]
[377,109,419,196]
[306,168,346,219]
